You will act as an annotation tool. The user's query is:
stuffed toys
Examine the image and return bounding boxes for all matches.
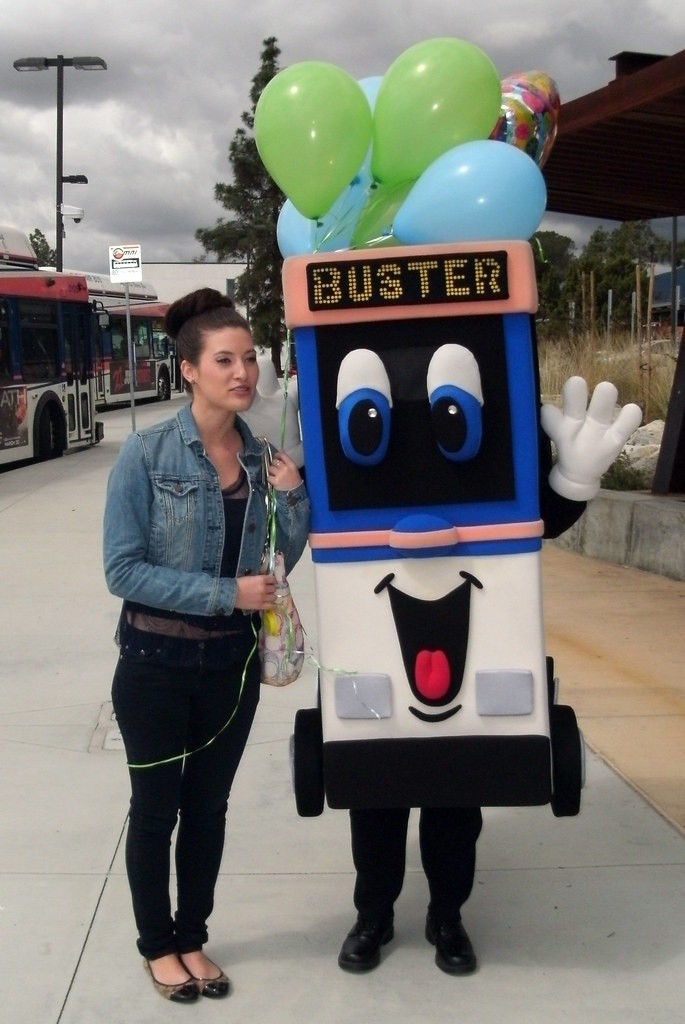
[236,241,642,977]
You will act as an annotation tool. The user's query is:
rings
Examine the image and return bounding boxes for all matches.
[275,460,282,467]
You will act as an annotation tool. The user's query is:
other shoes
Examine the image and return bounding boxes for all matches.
[142,957,199,1002]
[178,952,230,996]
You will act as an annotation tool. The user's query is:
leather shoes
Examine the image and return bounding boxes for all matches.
[338,917,394,971]
[426,909,475,974]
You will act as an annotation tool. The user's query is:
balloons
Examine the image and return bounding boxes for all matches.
[276,186,365,256]
[254,61,373,229]
[492,71,561,170]
[351,76,384,186]
[394,139,547,246]
[369,37,502,189]
[350,179,418,249]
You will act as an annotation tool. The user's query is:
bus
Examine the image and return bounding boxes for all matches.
[0,225,105,466]
[37,265,186,408]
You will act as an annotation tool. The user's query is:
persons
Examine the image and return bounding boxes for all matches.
[103,288,310,1003]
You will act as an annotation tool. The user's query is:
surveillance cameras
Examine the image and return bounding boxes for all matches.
[64,207,84,223]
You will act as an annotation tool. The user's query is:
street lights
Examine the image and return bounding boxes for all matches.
[11,54,108,273]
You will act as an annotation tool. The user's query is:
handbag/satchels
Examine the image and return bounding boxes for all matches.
[257,436,304,687]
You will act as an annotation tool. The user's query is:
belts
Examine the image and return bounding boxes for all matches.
[126,612,238,639]
